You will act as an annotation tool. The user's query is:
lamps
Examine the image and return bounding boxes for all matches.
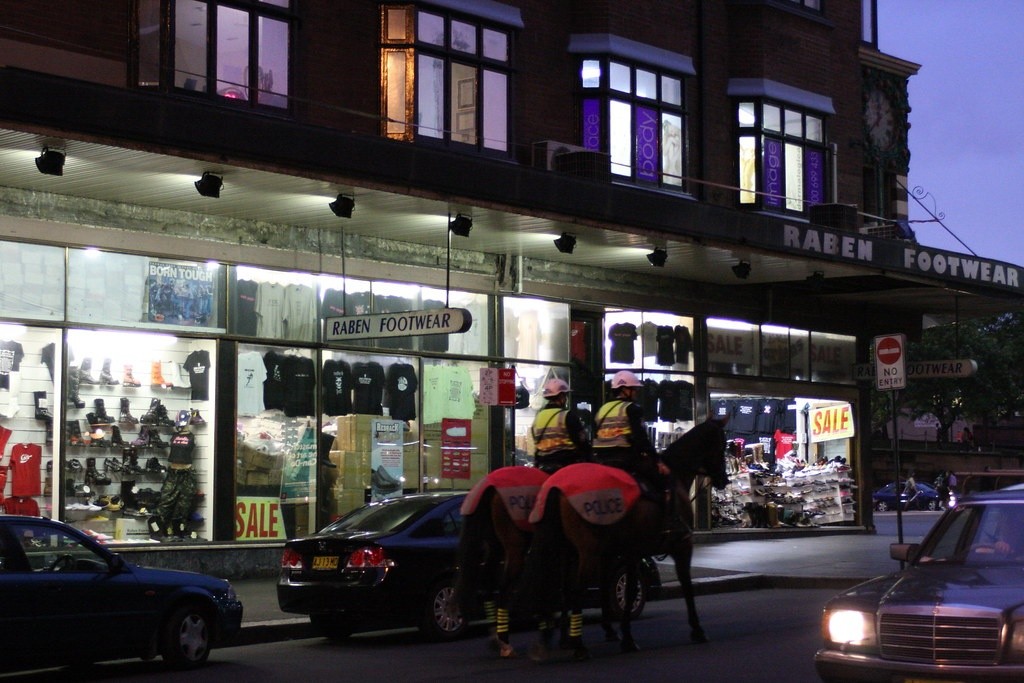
[328,194,354,218]
[732,259,752,278]
[646,246,668,267]
[450,214,472,237]
[195,171,223,198]
[35,143,67,176]
[806,271,825,288]
[554,232,576,254]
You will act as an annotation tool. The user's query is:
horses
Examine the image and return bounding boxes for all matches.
[462,411,731,664]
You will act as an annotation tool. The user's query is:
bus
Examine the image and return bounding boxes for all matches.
[937,469,1024,495]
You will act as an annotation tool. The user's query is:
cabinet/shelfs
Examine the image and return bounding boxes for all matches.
[65,422,198,523]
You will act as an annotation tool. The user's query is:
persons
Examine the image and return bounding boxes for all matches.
[531,376,592,475]
[903,470,922,510]
[934,469,958,510]
[151,408,197,541]
[960,426,973,444]
[993,518,1024,554]
[589,367,681,532]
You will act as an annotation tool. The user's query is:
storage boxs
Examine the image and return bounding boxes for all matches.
[238,413,489,537]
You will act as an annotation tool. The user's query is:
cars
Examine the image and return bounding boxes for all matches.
[276,491,662,643]
[872,481,962,513]
[0,514,244,671]
[813,489,1024,683]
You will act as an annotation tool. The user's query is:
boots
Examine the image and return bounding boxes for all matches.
[34,342,206,524]
[322,432,337,467]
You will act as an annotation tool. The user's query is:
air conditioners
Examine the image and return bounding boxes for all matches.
[531,140,586,173]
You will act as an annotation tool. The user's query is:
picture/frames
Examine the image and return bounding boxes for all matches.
[455,109,476,133]
[455,77,476,111]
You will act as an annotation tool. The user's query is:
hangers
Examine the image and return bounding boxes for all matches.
[22,434,32,446]
[294,348,303,356]
[718,394,774,403]
[194,341,202,351]
[332,352,340,361]
[360,356,370,363]
[397,356,404,364]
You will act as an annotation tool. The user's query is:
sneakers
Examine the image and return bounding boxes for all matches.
[710,438,857,530]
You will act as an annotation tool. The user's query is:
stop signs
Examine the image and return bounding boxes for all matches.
[876,338,902,365]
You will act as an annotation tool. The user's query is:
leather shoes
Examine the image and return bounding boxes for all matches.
[370,465,401,494]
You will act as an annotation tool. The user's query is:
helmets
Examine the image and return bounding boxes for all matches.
[611,370,645,388]
[543,378,574,398]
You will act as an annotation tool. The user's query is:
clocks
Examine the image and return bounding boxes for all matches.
[863,77,904,154]
